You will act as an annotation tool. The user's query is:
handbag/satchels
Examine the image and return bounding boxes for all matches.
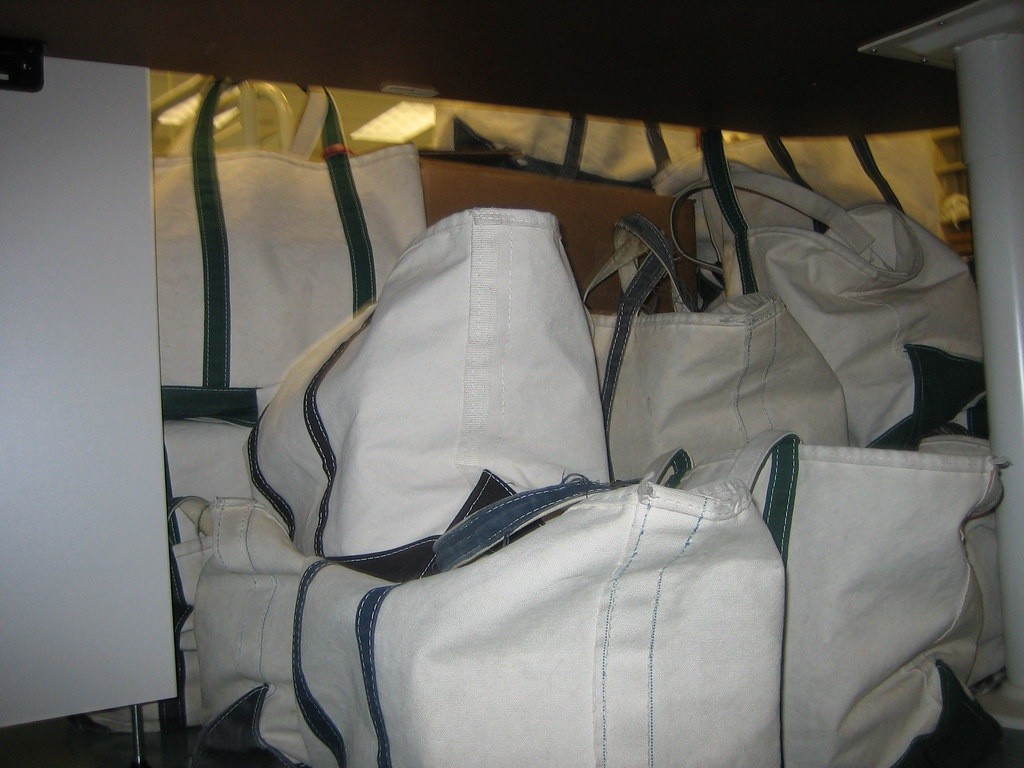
[73,78,1009,768]
